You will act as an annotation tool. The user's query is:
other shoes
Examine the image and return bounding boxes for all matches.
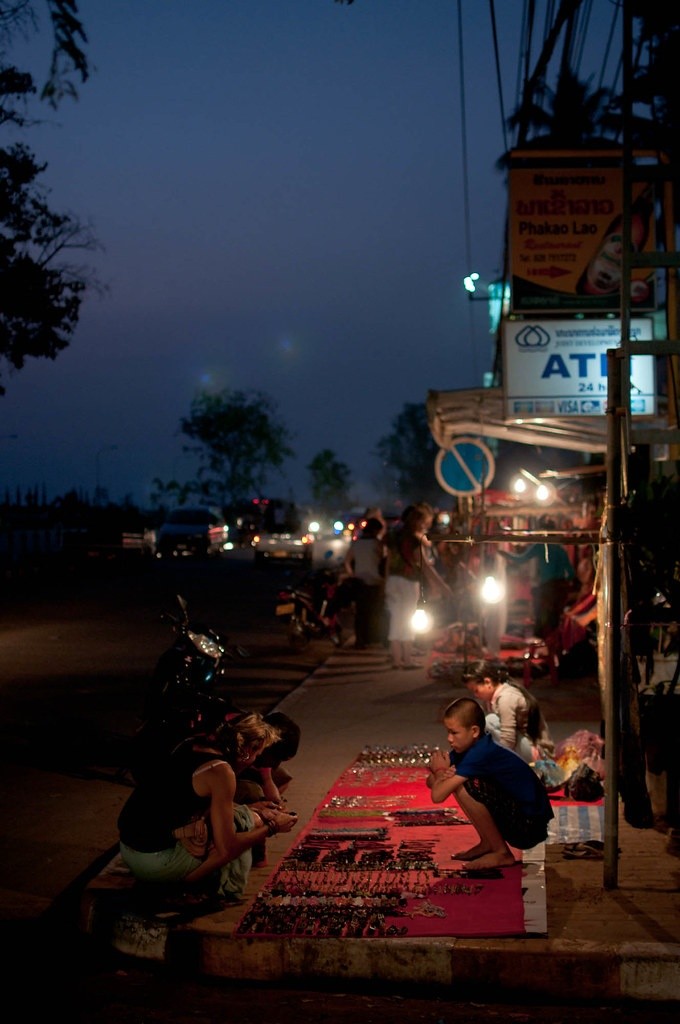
[665,826,680,848]
[404,663,422,670]
[391,663,399,669]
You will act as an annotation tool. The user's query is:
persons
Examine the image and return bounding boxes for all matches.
[461,660,550,764]
[234,712,302,867]
[426,698,555,870]
[118,710,298,906]
[338,502,574,669]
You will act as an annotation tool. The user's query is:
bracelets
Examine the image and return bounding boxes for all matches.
[265,818,280,835]
[434,767,446,772]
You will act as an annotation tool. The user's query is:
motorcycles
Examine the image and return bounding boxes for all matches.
[139,594,249,739]
[275,549,352,656]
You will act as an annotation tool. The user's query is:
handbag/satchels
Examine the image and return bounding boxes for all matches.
[564,764,605,801]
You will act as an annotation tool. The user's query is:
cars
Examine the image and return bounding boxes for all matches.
[155,496,317,572]
